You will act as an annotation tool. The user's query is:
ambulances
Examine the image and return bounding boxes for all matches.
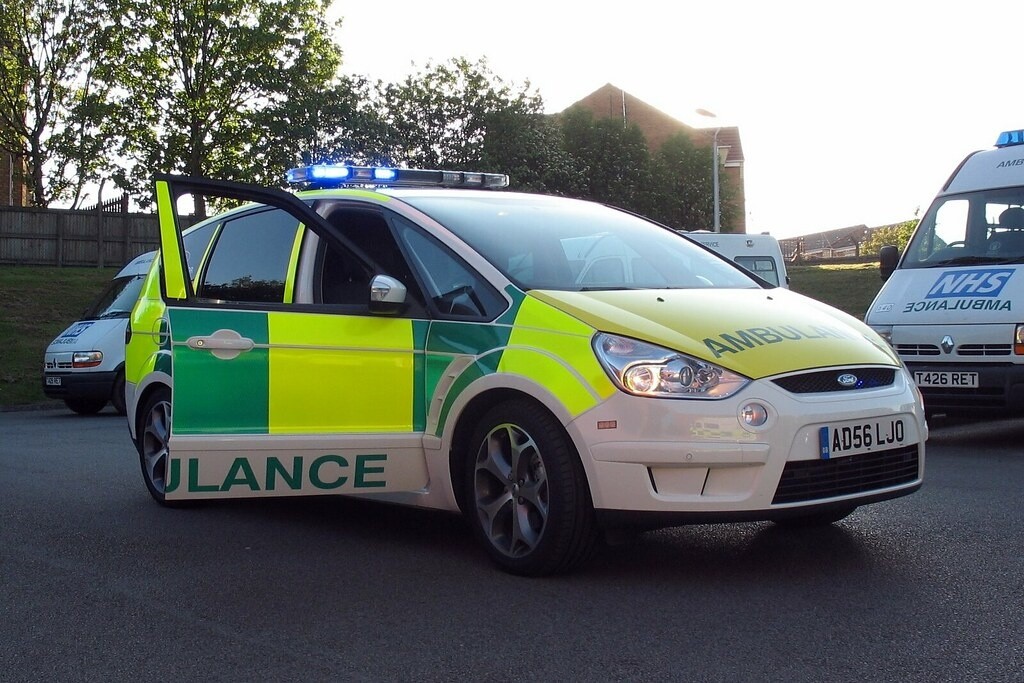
[40,247,159,415]
[509,230,789,291]
[864,129,1024,420]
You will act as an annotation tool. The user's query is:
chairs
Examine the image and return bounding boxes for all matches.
[985,207,1024,258]
[326,235,393,304]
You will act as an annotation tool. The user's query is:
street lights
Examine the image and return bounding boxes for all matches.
[696,108,724,233]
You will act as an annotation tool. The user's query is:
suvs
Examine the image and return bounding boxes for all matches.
[123,164,928,577]
